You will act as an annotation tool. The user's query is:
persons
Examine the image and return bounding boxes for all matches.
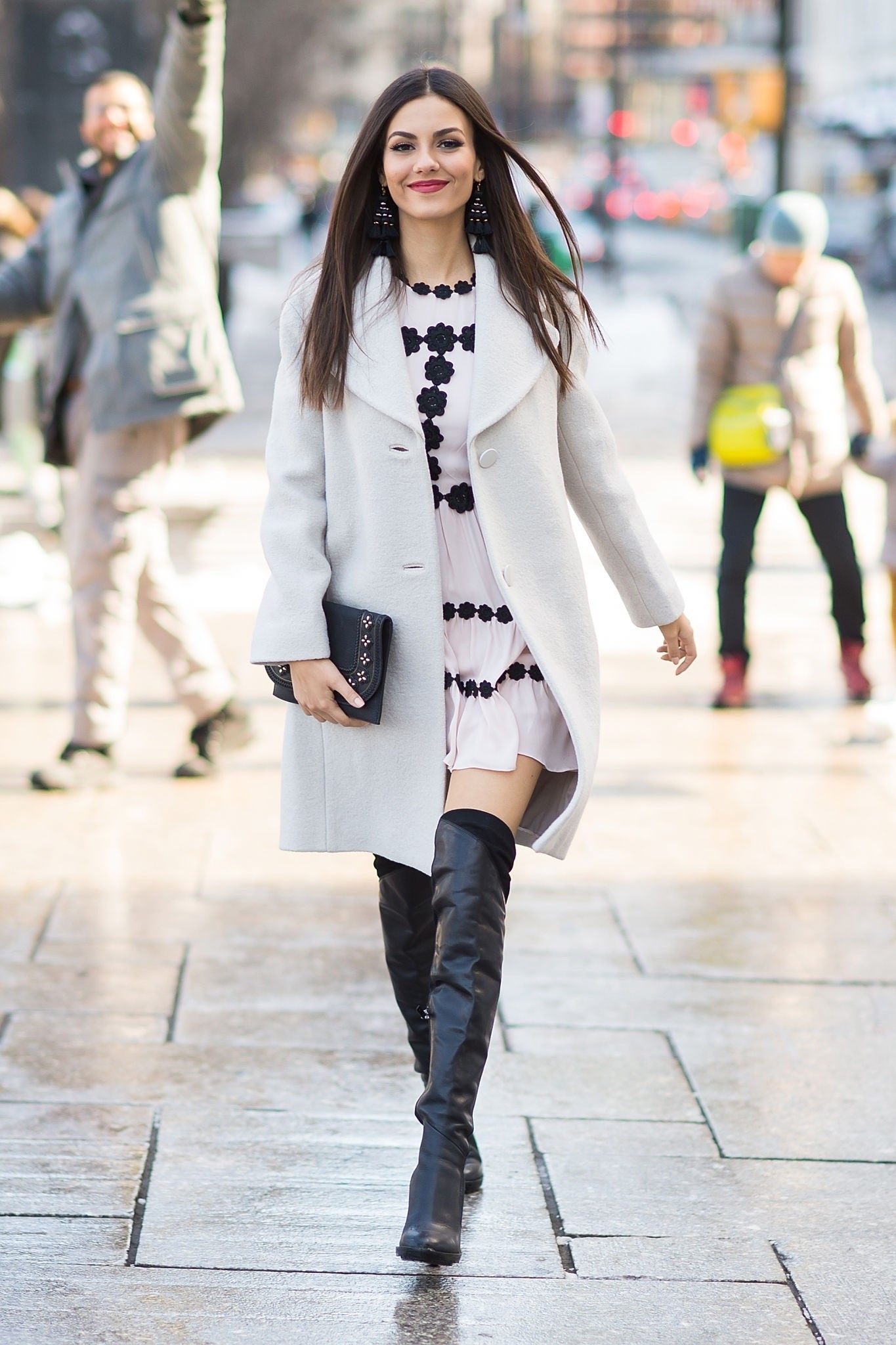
[247,68,697,1265]
[850,389,896,657]
[0,0,260,791]
[690,193,889,709]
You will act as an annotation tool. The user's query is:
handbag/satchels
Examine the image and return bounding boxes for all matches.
[709,383,794,473]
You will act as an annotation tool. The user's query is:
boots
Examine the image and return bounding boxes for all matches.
[371,809,519,1268]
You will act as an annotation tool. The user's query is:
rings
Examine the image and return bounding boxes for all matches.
[678,647,686,652]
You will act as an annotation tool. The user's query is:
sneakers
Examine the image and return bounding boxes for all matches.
[708,649,749,708]
[33,749,129,796]
[173,703,251,777]
[832,644,873,706]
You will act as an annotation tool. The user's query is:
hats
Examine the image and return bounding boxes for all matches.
[759,187,834,251]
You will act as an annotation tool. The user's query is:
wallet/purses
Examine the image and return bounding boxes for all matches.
[251,599,390,725]
